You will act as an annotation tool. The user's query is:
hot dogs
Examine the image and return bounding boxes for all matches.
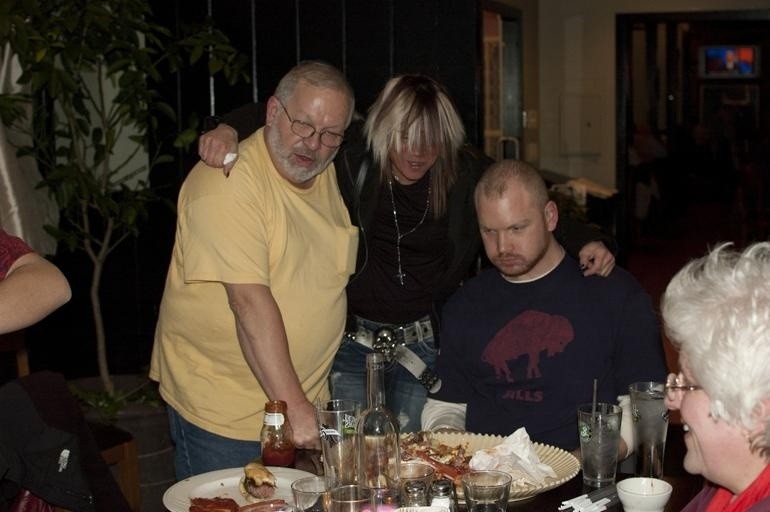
[238,461,279,502]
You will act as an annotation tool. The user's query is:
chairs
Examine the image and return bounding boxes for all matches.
[0,331,141,512]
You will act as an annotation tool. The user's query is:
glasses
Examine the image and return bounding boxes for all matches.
[664,370,704,401]
[276,96,346,148]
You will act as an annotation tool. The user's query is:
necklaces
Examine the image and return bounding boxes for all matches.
[385,169,432,286]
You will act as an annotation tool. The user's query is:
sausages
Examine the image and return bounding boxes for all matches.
[240,499,286,511]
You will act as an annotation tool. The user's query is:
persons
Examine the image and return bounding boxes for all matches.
[1,224,75,337]
[654,240,770,512]
[437,158,669,462]
[198,72,617,436]
[149,56,362,483]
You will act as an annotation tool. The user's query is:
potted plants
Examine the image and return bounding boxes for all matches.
[0,0,251,512]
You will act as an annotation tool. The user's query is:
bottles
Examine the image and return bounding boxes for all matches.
[352,352,402,500]
[259,399,295,469]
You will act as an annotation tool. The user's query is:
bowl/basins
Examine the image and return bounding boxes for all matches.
[617,476,673,511]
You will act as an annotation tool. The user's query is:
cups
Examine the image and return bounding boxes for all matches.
[576,403,622,487]
[628,381,671,483]
[313,398,364,491]
[291,463,512,512]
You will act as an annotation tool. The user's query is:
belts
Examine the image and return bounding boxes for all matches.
[345,319,442,394]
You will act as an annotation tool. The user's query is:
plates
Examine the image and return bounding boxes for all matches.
[162,466,322,512]
[193,466,322,512]
[320,430,582,505]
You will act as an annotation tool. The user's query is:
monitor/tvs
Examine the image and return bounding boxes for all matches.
[699,44,761,80]
